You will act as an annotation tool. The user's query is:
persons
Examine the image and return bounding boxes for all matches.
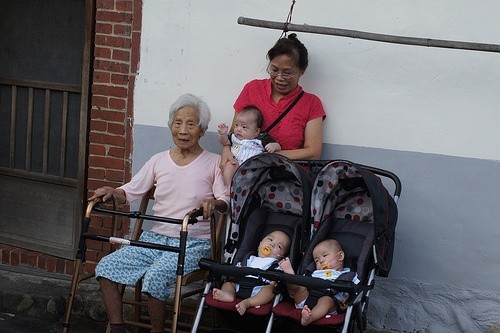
[218,32,327,182]
[277,239,351,326]
[86,93,232,333]
[218,104,282,198]
[212,230,292,316]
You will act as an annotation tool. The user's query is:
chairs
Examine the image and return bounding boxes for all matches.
[106,186,227,333]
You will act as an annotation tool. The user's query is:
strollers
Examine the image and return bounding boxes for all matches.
[190,152,403,333]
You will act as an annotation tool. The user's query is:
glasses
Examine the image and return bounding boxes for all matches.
[266,64,302,80]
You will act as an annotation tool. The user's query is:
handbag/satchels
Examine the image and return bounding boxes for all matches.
[257,130,273,147]
